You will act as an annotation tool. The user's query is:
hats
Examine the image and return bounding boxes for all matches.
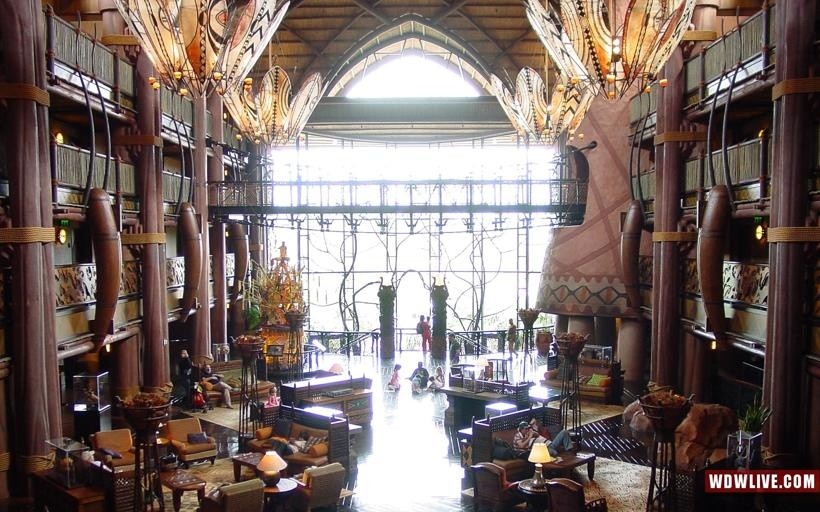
[518,421,529,430]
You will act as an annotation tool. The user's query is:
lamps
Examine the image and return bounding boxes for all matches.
[487,43,599,149]
[114,0,291,104]
[524,2,696,111]
[217,19,331,149]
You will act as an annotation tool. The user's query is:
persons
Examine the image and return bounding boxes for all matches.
[506,319,519,360]
[408,361,429,393]
[426,366,444,394]
[513,420,536,473]
[264,386,278,409]
[448,333,461,365]
[422,317,432,351]
[202,363,242,409]
[528,423,574,457]
[272,429,309,458]
[416,315,425,352]
[178,349,194,400]
[387,364,402,391]
[682,392,696,423]
